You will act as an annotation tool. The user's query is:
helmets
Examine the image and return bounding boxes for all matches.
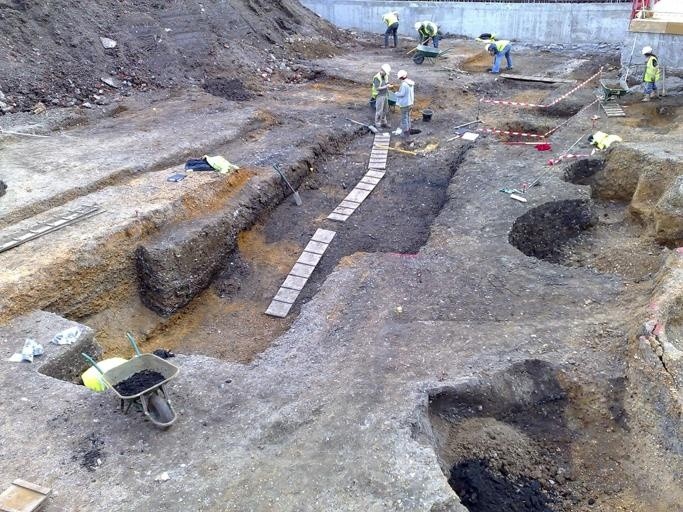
[382,64,391,75]
[485,44,491,52]
[414,22,421,30]
[642,46,652,55]
[397,70,407,79]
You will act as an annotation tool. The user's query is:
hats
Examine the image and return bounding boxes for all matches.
[641,91,656,102]
[423,21,437,36]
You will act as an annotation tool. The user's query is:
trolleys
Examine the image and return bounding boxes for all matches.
[369,90,398,113]
[411,43,454,65]
[598,66,629,107]
[79,333,179,433]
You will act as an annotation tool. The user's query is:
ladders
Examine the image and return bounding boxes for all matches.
[596,95,626,117]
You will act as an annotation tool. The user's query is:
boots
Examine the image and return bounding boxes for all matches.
[399,128,411,139]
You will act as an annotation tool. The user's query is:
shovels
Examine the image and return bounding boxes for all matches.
[273,164,303,206]
[453,116,485,129]
[345,118,379,133]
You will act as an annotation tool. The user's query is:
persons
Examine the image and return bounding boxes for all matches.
[484,39,512,74]
[382,11,440,49]
[640,46,660,104]
[370,63,414,143]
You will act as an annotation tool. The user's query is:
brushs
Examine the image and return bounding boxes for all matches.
[510,134,586,203]
